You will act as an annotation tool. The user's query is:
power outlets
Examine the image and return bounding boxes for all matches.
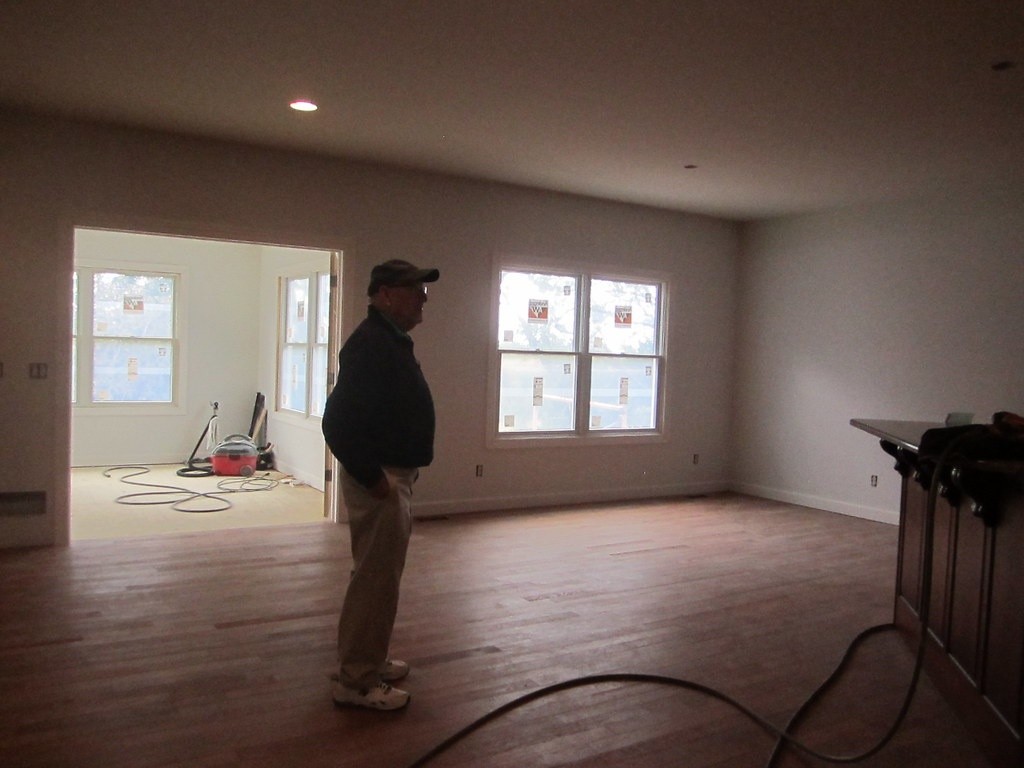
[870,475,877,486]
[476,465,484,477]
[694,454,698,464]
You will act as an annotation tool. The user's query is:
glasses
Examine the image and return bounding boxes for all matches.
[388,282,429,295]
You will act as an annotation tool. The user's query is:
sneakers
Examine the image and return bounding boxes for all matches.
[332,682,411,712]
[326,655,410,684]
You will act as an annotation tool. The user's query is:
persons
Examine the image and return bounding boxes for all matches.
[322,260,439,711]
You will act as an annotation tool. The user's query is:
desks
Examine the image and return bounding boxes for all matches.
[848,419,1024,768]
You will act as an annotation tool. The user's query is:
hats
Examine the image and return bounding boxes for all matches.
[367,259,440,298]
